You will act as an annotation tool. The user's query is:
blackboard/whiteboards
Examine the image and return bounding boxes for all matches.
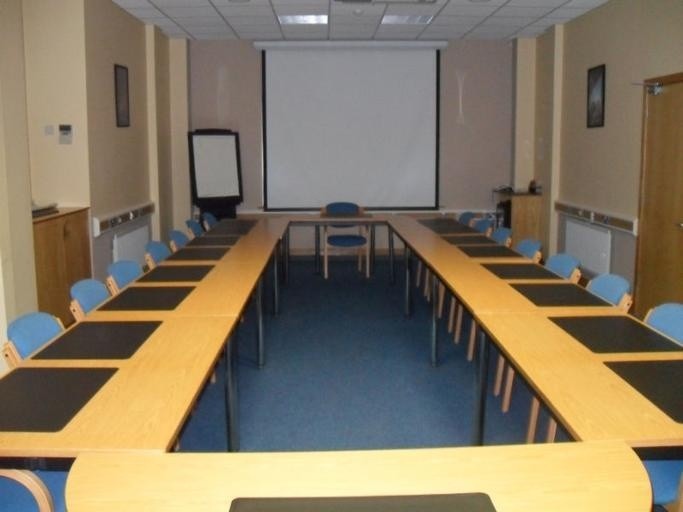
[188,39,516,214]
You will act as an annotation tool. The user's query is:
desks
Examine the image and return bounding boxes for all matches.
[55,436,658,512]
[490,184,544,264]
[279,212,398,292]
[379,210,683,456]
[0,212,295,460]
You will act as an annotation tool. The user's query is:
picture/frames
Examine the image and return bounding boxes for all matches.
[585,62,608,128]
[112,62,130,129]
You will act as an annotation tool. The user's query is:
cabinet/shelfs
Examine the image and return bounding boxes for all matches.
[30,203,96,331]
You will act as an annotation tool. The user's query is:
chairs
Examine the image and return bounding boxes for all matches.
[168,231,188,254]
[204,215,218,233]
[500,302,683,442]
[1,312,65,370]
[69,278,111,322]
[413,208,513,311]
[1,458,74,510]
[184,220,201,239]
[453,255,582,362]
[437,238,543,333]
[143,240,172,271]
[547,407,681,509]
[475,273,635,396]
[107,260,143,297]
[311,202,372,281]
